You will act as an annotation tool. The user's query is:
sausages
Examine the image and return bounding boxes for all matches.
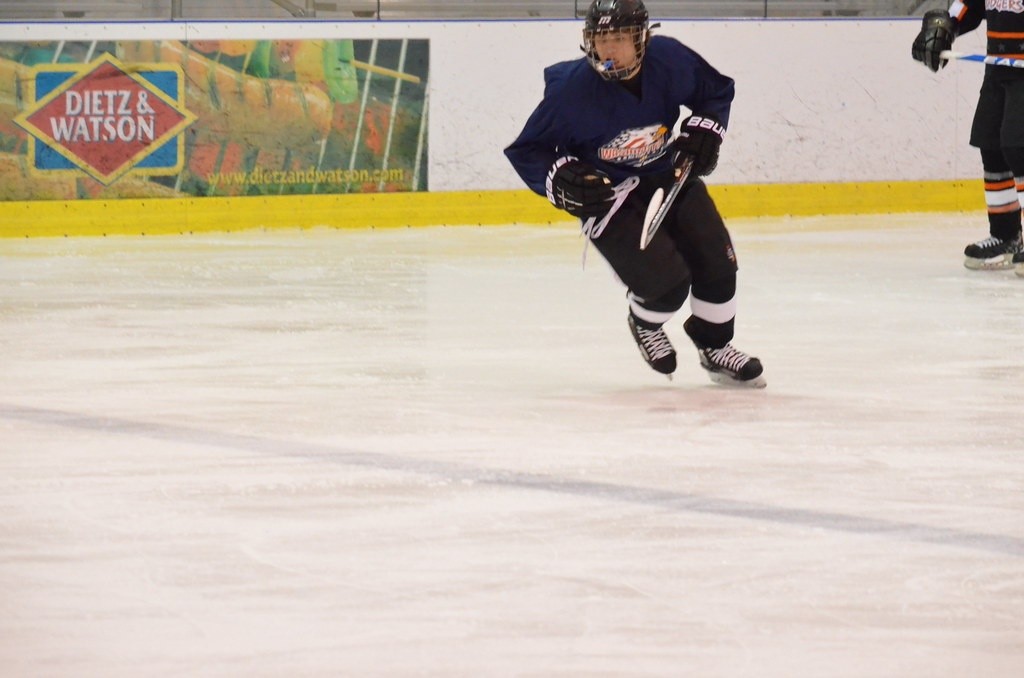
[0,40,329,202]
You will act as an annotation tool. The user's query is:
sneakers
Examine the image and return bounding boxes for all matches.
[699,343,768,388]
[628,304,677,382]
[965,233,1024,276]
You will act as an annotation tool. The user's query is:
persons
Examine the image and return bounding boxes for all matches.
[502,0,766,389]
[912,0,1024,279]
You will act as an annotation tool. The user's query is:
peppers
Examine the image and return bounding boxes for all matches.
[324,39,359,102]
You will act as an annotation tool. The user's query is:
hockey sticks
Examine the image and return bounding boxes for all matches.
[938,49,1024,69]
[639,157,695,251]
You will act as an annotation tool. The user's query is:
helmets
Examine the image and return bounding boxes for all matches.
[580,0,661,80]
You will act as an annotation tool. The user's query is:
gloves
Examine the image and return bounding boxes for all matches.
[546,151,620,218]
[670,130,724,191]
[912,9,959,73]
